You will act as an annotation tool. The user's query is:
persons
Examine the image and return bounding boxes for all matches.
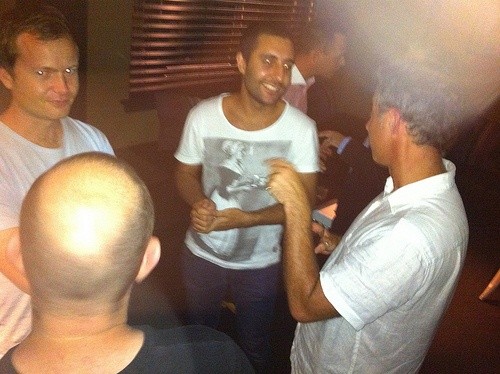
[172,24,317,373]
[284,18,346,175]
[318,126,390,236]
[0,4,118,360]
[0,151,256,374]
[263,58,470,374]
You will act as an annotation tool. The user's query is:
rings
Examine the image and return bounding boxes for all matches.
[321,239,330,249]
